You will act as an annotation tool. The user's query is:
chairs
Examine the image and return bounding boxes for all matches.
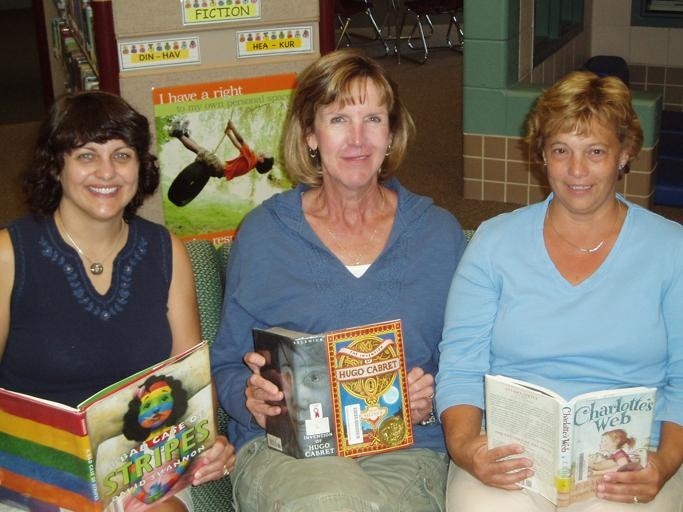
[332,0,463,67]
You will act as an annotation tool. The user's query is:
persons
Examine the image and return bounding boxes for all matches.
[275,338,332,456]
[209,45,468,511]
[169,114,274,180]
[431,69,682,511]
[0,91,239,512]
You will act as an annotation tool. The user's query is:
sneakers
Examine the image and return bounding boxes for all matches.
[170,119,181,137]
[181,119,191,136]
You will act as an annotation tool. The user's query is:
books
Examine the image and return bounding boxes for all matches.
[482,373,656,507]
[0,340,216,510]
[251,318,414,458]
[47,0,98,93]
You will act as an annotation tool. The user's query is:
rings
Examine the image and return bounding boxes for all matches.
[222,464,230,476]
[631,493,640,505]
[426,391,436,399]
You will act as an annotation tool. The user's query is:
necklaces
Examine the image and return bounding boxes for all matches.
[314,209,384,266]
[57,206,125,275]
[546,206,620,253]
